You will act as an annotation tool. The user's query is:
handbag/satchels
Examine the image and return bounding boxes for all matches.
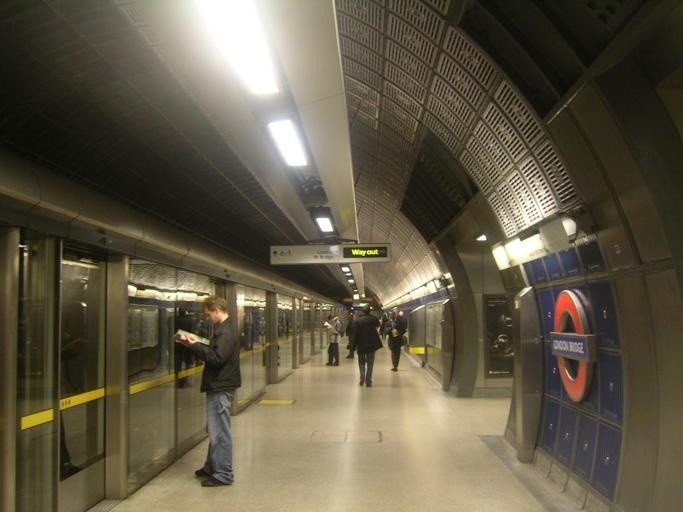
[390,333,407,348]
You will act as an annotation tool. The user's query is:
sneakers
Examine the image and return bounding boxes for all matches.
[194,468,228,486]
[359,376,372,387]
[325,351,354,366]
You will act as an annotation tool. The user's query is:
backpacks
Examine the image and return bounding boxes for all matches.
[335,318,344,337]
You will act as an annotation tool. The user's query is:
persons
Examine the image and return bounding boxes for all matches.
[349,303,384,388]
[386,312,407,372]
[379,313,387,341]
[324,313,341,366]
[396,310,407,336]
[184,294,242,487]
[343,313,354,359]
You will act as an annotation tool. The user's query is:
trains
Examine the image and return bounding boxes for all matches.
[0,144,350,512]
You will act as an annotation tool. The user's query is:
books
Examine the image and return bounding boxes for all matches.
[173,329,210,348]
